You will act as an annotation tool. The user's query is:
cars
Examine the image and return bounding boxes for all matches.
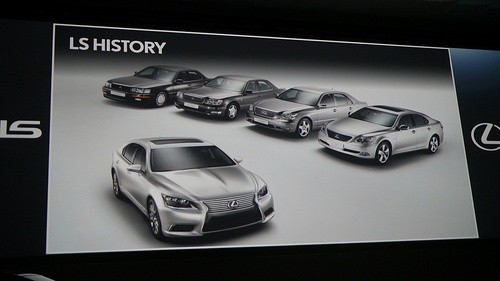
[110,137,275,242]
[102,65,214,109]
[246,85,368,139]
[317,105,444,166]
[174,74,288,121]
